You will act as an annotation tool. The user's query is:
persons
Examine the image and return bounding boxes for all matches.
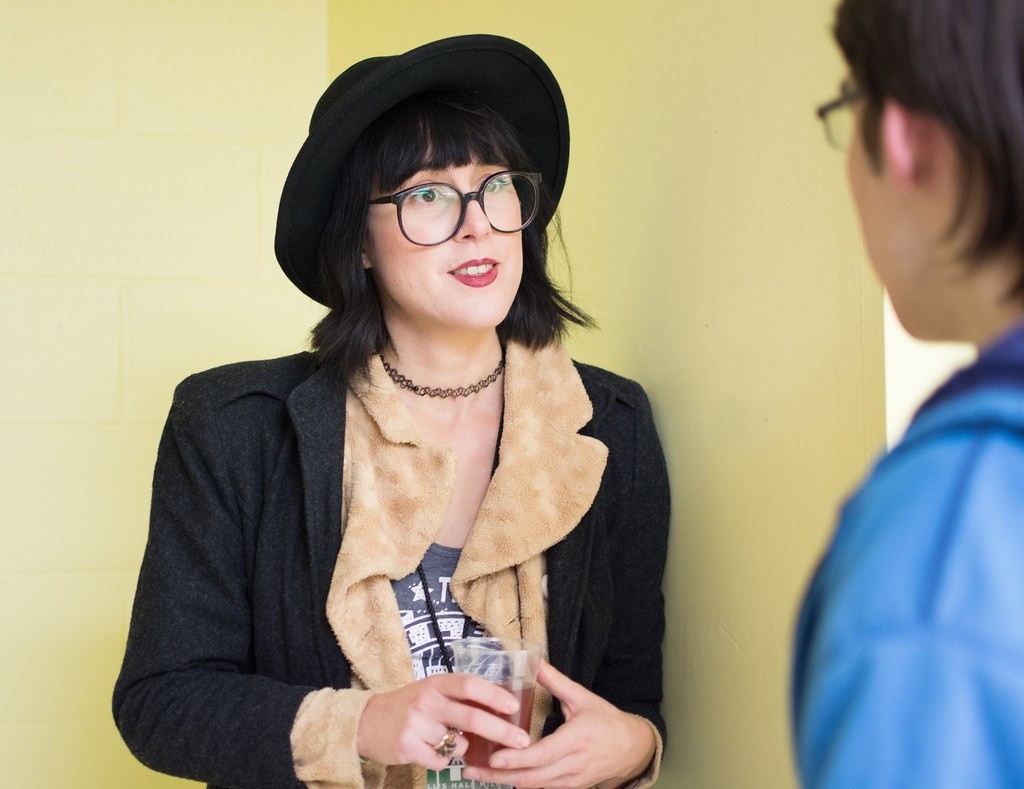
[112,35,672,789]
[790,1,1024,789]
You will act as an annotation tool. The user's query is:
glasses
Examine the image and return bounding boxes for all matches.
[818,84,873,152]
[367,169,540,248]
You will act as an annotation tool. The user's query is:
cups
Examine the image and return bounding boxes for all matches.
[451,637,542,768]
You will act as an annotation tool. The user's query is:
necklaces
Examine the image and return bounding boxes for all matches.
[379,355,503,399]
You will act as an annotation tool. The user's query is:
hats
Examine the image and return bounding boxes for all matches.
[272,34,571,308]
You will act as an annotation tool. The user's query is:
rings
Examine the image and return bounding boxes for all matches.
[431,730,457,758]
